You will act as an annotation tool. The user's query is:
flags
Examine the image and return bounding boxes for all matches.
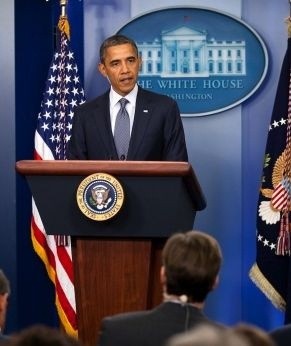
[248,18,291,313]
[30,0,88,342]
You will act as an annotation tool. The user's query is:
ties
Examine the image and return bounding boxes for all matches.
[114,98,131,160]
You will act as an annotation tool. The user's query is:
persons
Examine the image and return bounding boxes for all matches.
[99,229,252,345]
[71,34,189,161]
[1,264,13,345]
